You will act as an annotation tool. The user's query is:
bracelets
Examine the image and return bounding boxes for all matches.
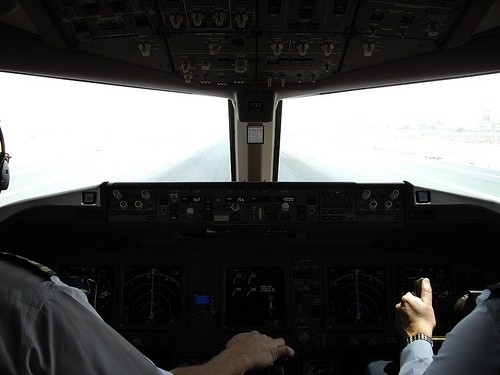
[403,332,433,346]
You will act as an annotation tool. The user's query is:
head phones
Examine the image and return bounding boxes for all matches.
[0,128,11,193]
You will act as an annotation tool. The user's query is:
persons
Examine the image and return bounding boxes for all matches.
[0,126,294,375]
[394,276,500,375]
